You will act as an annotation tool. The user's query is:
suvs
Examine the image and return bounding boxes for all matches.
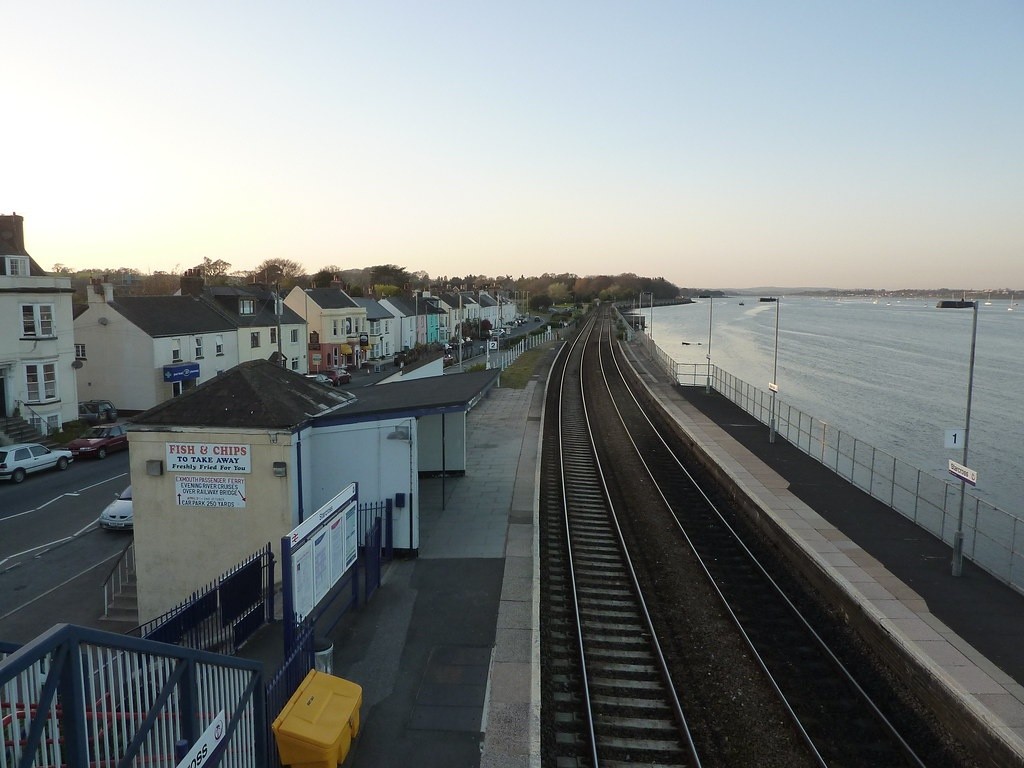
[78,399,118,425]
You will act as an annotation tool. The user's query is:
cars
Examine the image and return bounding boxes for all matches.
[535,316,540,322]
[99,485,133,531]
[303,369,352,389]
[65,421,132,460]
[442,337,473,367]
[507,316,528,328]
[0,443,75,485]
[492,329,506,338]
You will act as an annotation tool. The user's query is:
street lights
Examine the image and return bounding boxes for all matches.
[304,288,313,374]
[459,290,475,373]
[758,297,779,444]
[643,292,653,362]
[937,298,979,577]
[697,294,712,393]
[514,290,531,315]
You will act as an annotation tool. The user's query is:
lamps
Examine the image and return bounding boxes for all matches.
[71,359,84,369]
[97,318,109,326]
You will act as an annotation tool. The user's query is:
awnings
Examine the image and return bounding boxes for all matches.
[341,344,352,354]
[363,344,372,350]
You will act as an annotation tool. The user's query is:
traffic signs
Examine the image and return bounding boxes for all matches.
[176,474,246,508]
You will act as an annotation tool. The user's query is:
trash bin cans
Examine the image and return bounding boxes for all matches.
[270,668,362,768]
[314,639,333,675]
